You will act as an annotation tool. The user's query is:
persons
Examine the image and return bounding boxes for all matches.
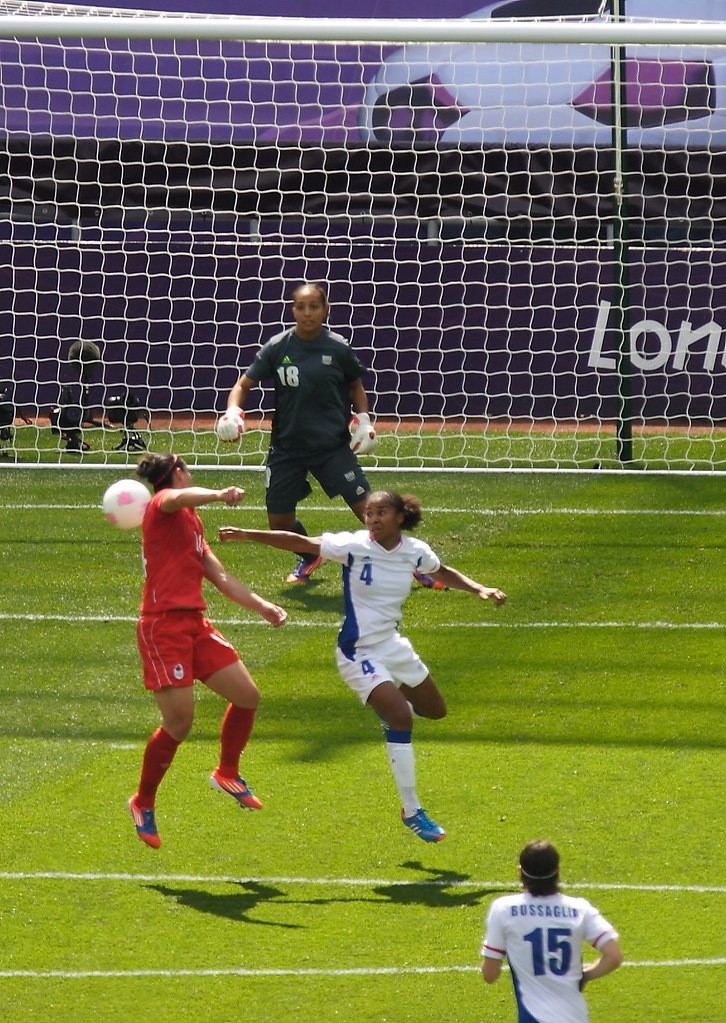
[480,840,624,1023]
[219,488,508,843]
[217,283,447,590]
[130,453,288,849]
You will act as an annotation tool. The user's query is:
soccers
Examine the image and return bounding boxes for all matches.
[102,479,151,531]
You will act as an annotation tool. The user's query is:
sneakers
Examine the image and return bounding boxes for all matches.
[129,794,159,849]
[210,766,261,810]
[413,571,450,590]
[287,555,323,586]
[401,807,446,842]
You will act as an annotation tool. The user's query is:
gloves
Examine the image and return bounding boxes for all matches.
[216,406,245,441]
[349,411,377,455]
